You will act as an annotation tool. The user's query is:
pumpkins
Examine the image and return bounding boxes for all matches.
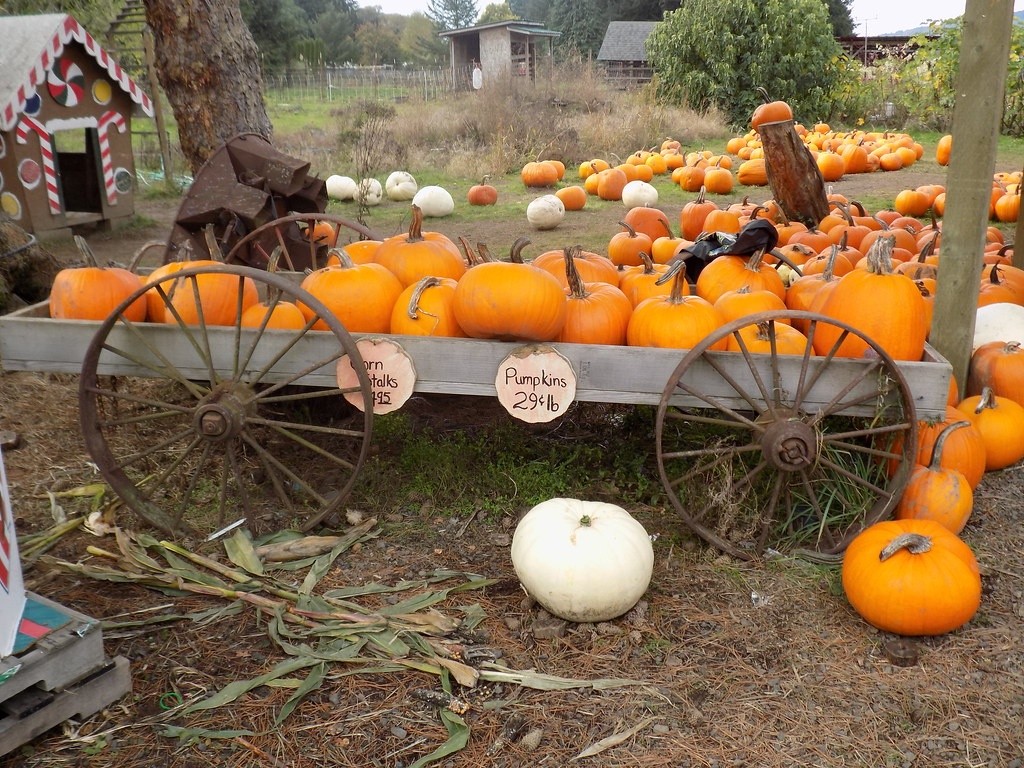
[49,86,1024,535]
[843,519,982,635]
[511,498,654,623]
